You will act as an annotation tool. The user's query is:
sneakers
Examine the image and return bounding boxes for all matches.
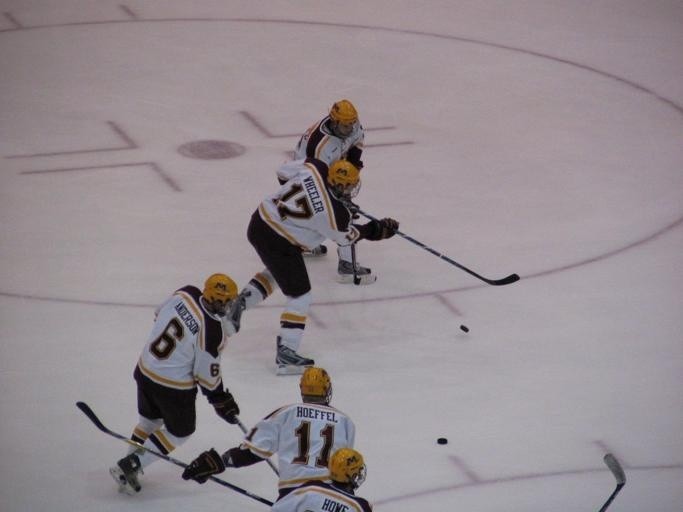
[302,244,327,257]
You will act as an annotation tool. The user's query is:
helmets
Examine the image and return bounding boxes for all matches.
[329,449,367,489]
[300,368,332,405]
[329,100,358,138]
[327,160,361,198]
[203,274,237,317]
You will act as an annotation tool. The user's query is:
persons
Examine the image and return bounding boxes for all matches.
[290,98,371,276]
[179,369,358,496]
[265,447,373,512]
[114,273,239,492]
[222,157,398,366]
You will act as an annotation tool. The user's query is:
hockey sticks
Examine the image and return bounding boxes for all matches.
[76,402,273,505]
[349,211,379,284]
[592,440,626,511]
[348,203,520,286]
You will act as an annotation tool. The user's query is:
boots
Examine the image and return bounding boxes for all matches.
[117,453,144,493]
[275,345,314,367]
[222,294,246,337]
[338,260,371,276]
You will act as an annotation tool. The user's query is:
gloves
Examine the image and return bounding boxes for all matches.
[380,218,399,238]
[212,394,239,424]
[182,448,225,484]
